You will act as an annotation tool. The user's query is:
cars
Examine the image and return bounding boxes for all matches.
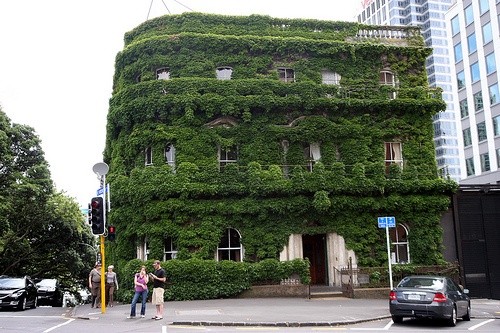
[0,276,37,310]
[389,276,471,325]
[33,278,64,306]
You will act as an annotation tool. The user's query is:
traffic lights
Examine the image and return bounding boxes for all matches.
[109,227,116,240]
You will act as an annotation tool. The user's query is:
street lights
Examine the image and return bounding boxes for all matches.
[91,197,104,235]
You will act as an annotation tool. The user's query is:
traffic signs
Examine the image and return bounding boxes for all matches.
[378,216,396,228]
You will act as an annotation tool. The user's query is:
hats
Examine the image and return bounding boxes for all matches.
[153,260,160,265]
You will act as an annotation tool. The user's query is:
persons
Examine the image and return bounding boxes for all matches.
[88,261,102,308]
[149,260,166,320]
[127,266,149,319]
[105,265,119,309]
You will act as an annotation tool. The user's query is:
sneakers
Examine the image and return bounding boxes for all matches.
[142,313,146,318]
[152,315,157,318]
[127,316,136,318]
[156,317,163,320]
